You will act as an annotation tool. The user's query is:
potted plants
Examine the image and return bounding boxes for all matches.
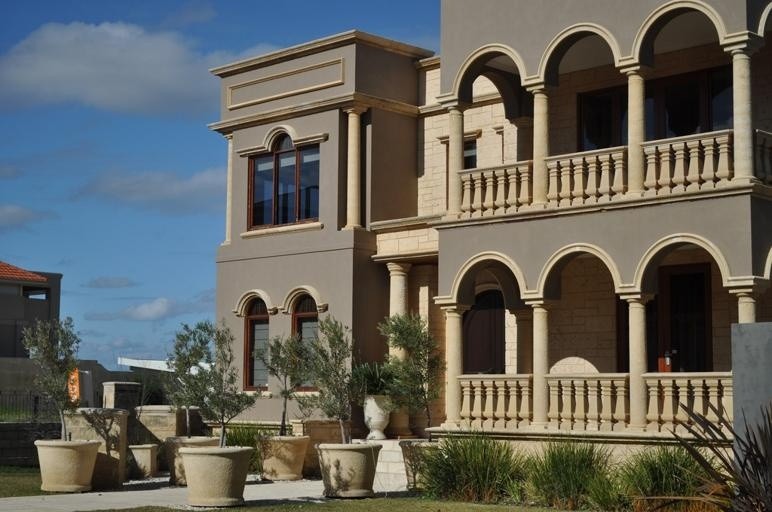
[28,316,107,493]
[118,377,159,481]
